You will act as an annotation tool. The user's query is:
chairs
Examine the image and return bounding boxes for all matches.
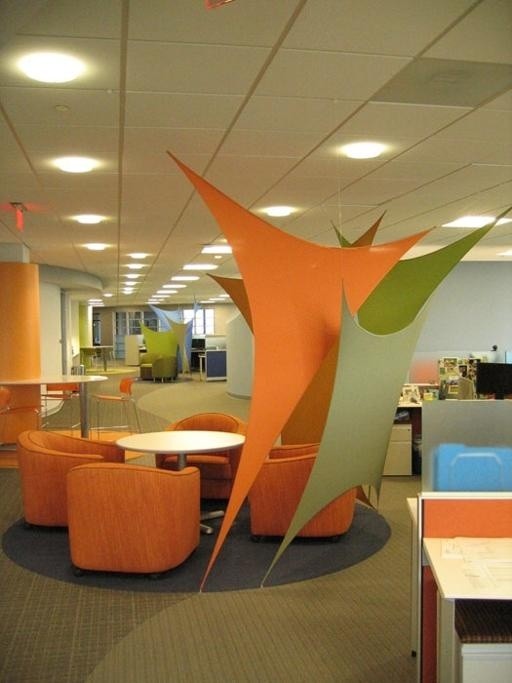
[0,373,136,442]
[137,343,226,383]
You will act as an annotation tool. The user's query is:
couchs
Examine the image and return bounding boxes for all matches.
[252,442,357,544]
[17,429,125,528]
[155,413,247,500]
[66,463,201,580]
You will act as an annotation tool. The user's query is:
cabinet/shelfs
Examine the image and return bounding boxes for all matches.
[381,372,512,477]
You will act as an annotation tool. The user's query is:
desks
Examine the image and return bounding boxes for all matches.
[115,430,245,535]
[80,345,115,372]
[406,491,511,683]
